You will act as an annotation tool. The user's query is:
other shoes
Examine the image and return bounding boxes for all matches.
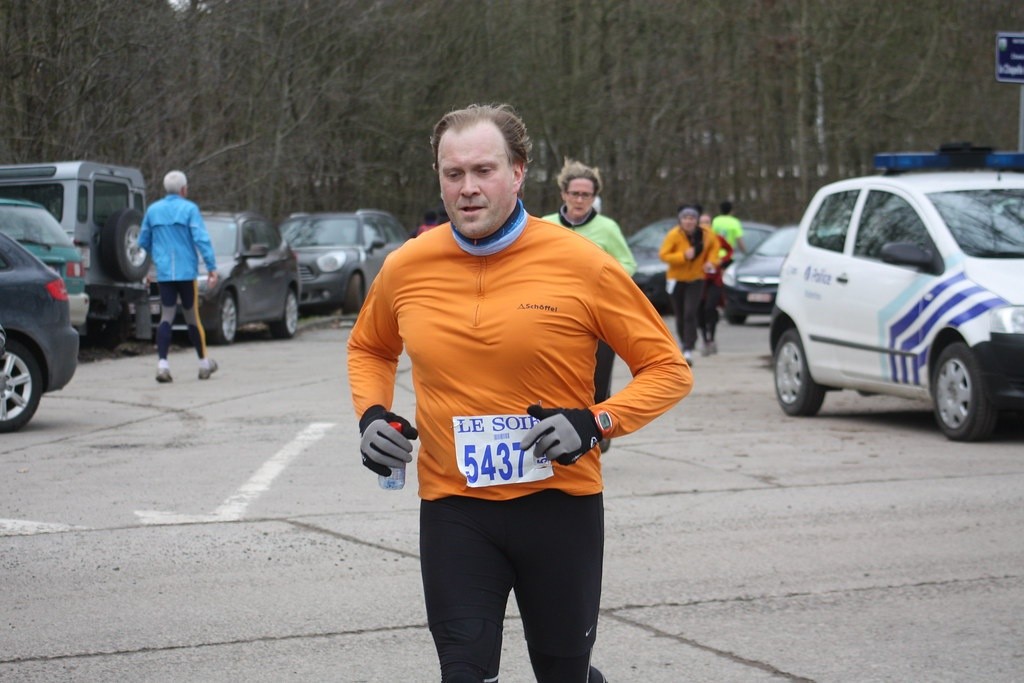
[198,358,217,379]
[156,367,173,383]
[700,344,717,356]
[683,347,692,366]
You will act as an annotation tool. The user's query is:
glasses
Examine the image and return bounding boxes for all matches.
[566,190,594,200]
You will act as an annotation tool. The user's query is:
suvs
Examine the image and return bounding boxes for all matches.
[0,162,150,346]
[279,209,411,314]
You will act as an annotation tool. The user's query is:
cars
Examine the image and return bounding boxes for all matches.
[0,198,89,329]
[0,232,83,432]
[722,224,842,325]
[130,208,301,340]
[626,214,776,311]
[768,151,1024,444]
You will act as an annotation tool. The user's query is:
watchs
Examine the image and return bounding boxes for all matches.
[592,410,613,437]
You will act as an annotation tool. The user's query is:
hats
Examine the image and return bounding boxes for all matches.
[677,205,701,223]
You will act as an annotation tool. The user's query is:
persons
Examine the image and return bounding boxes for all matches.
[140,169,217,382]
[699,214,735,357]
[417,212,439,236]
[711,201,748,311]
[347,105,692,683]
[659,205,718,367]
[541,162,639,455]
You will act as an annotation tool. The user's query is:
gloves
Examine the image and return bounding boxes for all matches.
[519,406,604,467]
[359,404,419,477]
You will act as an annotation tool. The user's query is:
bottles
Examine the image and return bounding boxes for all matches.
[379,422,407,489]
